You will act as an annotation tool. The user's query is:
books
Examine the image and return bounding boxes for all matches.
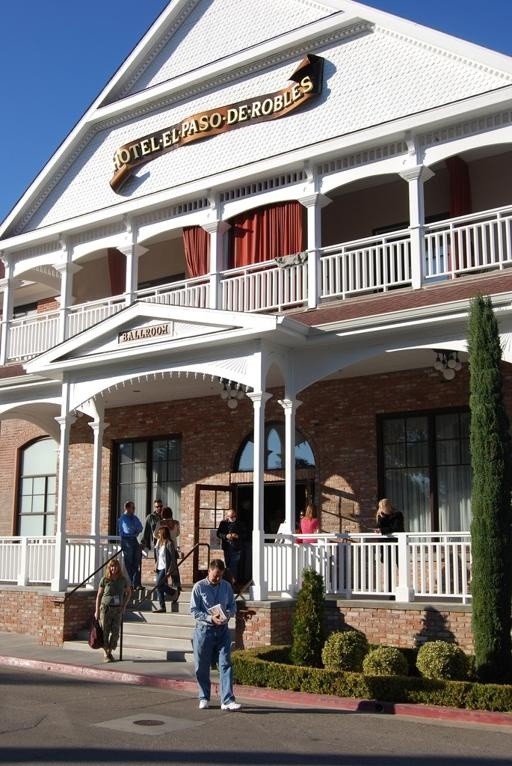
[207,603,229,620]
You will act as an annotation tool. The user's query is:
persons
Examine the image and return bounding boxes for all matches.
[153,525,180,612]
[216,509,243,580]
[152,506,180,592]
[298,504,320,543]
[190,559,242,710]
[94,559,132,663]
[140,499,164,556]
[117,500,145,591]
[375,498,405,601]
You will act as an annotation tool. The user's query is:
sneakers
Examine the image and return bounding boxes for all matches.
[102,653,117,664]
[199,697,211,711]
[220,699,242,714]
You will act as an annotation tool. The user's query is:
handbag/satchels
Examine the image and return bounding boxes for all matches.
[87,617,104,650]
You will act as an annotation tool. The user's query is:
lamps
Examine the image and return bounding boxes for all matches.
[218,377,245,409]
[433,349,464,381]
